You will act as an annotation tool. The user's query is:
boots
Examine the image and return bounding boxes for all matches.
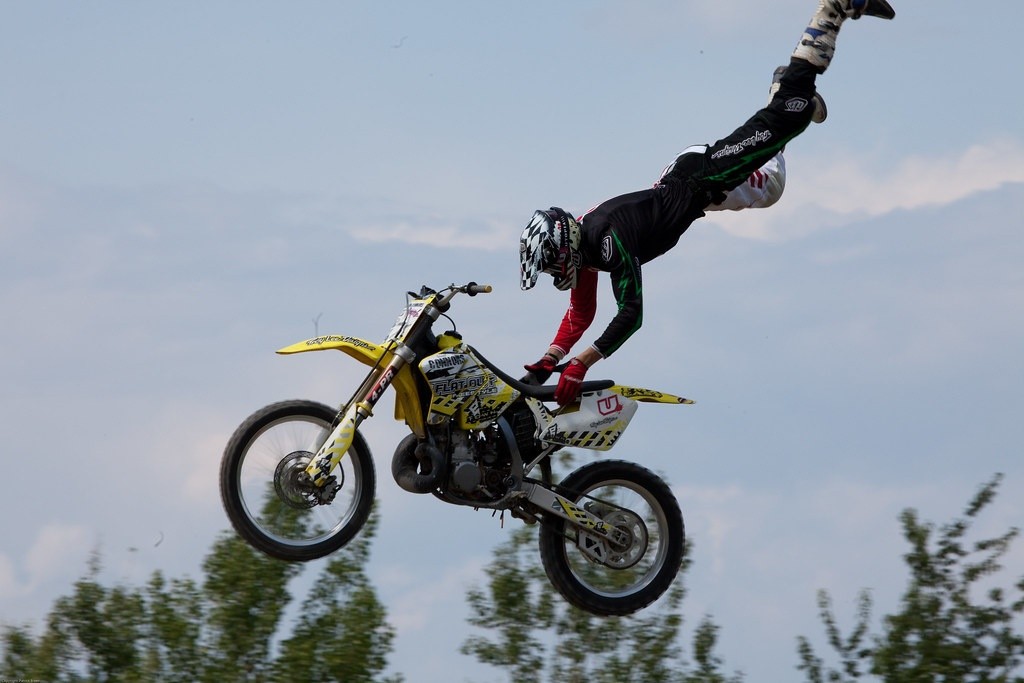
[792,0,895,74]
[768,66,827,123]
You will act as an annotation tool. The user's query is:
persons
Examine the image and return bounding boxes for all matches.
[517,0,896,409]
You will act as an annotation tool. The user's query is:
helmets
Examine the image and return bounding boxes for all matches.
[520,206,581,291]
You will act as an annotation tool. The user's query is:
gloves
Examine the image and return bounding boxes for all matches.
[543,357,588,405]
[524,352,559,384]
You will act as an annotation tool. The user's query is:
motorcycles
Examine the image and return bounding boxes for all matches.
[218,281,696,617]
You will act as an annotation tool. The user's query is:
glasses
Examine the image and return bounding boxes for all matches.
[543,249,568,278]
